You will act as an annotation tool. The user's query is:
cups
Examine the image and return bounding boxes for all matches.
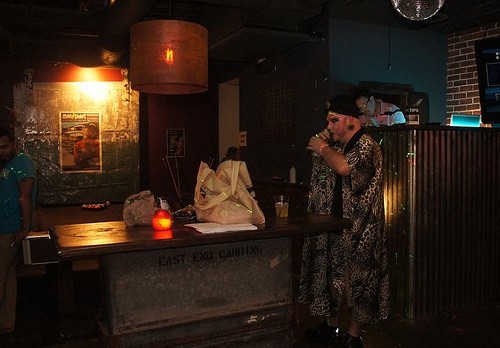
[273,196,289,218]
[311,136,323,157]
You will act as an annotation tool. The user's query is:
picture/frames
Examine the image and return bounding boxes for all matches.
[165,127,185,158]
[58,109,103,174]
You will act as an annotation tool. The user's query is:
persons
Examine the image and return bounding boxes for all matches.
[72,121,100,170]
[350,92,407,129]
[220,146,239,161]
[296,92,390,348]
[0,125,38,335]
[0,106,15,128]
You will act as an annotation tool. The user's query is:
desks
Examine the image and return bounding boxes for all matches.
[48,212,353,348]
[251,175,312,190]
[22,230,73,319]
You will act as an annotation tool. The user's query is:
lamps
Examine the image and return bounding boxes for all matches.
[150,209,172,232]
[126,0,209,95]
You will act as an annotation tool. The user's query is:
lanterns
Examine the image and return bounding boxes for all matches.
[128,18,210,96]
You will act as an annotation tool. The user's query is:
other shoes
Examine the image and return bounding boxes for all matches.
[338,333,364,348]
[304,320,340,345]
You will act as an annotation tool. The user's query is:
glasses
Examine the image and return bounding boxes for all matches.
[360,99,370,111]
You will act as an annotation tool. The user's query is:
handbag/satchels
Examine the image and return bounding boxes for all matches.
[195,160,266,225]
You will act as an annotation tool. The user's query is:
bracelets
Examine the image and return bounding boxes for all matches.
[319,142,329,155]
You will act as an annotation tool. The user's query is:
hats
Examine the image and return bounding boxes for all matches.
[324,94,361,119]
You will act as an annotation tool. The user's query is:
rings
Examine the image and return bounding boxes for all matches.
[316,132,320,137]
[324,128,328,132]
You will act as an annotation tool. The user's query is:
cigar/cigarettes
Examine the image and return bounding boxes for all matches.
[10,240,15,247]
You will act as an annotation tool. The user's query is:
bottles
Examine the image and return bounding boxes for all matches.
[289,165,296,183]
